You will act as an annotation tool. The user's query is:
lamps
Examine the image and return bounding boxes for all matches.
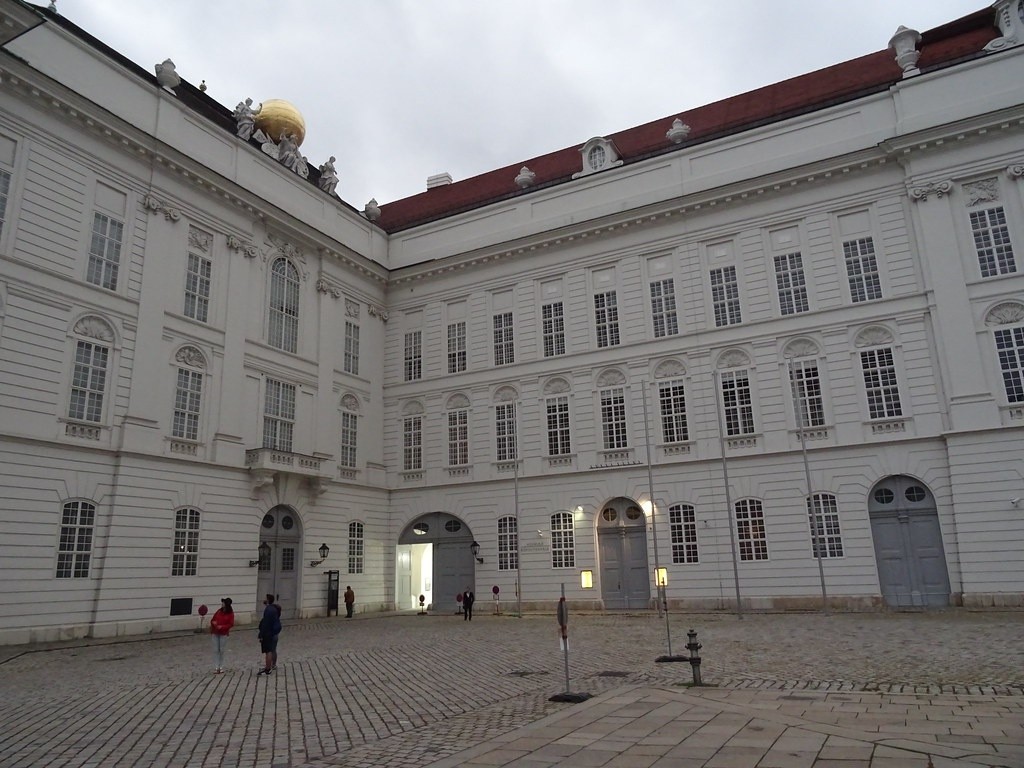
[654,567,668,586]
[685,629,704,658]
[310,543,330,567]
[470,541,483,564]
[250,541,270,568]
[538,529,543,537]
[580,570,593,589]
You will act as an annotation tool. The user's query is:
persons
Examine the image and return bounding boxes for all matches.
[967,182,997,207]
[257,593,282,676]
[210,598,234,674]
[344,585,354,618]
[277,124,310,181]
[319,155,341,200]
[463,586,474,622]
[232,97,263,143]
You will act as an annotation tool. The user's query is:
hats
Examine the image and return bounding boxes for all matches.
[266,594,274,603]
[221,598,232,606]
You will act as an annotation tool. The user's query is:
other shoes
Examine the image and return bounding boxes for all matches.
[272,664,277,670]
[213,667,220,674]
[219,667,224,673]
[255,668,273,676]
